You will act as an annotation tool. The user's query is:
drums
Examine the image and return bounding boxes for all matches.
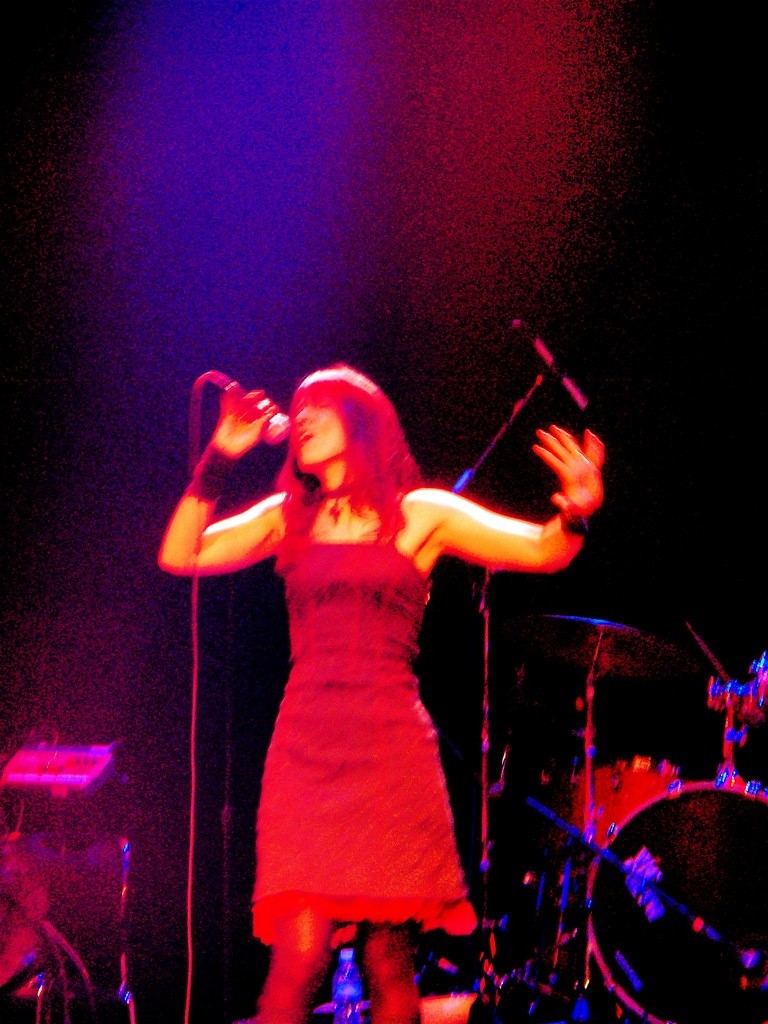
[586,777,768,1024]
[572,755,681,862]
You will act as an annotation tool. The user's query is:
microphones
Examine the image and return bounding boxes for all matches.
[517,319,588,412]
[742,949,766,976]
[210,370,291,445]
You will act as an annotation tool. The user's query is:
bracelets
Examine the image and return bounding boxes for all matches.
[186,444,236,503]
[554,508,594,541]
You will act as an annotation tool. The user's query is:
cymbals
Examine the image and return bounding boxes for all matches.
[524,612,700,678]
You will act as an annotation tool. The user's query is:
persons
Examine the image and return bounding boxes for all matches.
[157,362,605,1024]
